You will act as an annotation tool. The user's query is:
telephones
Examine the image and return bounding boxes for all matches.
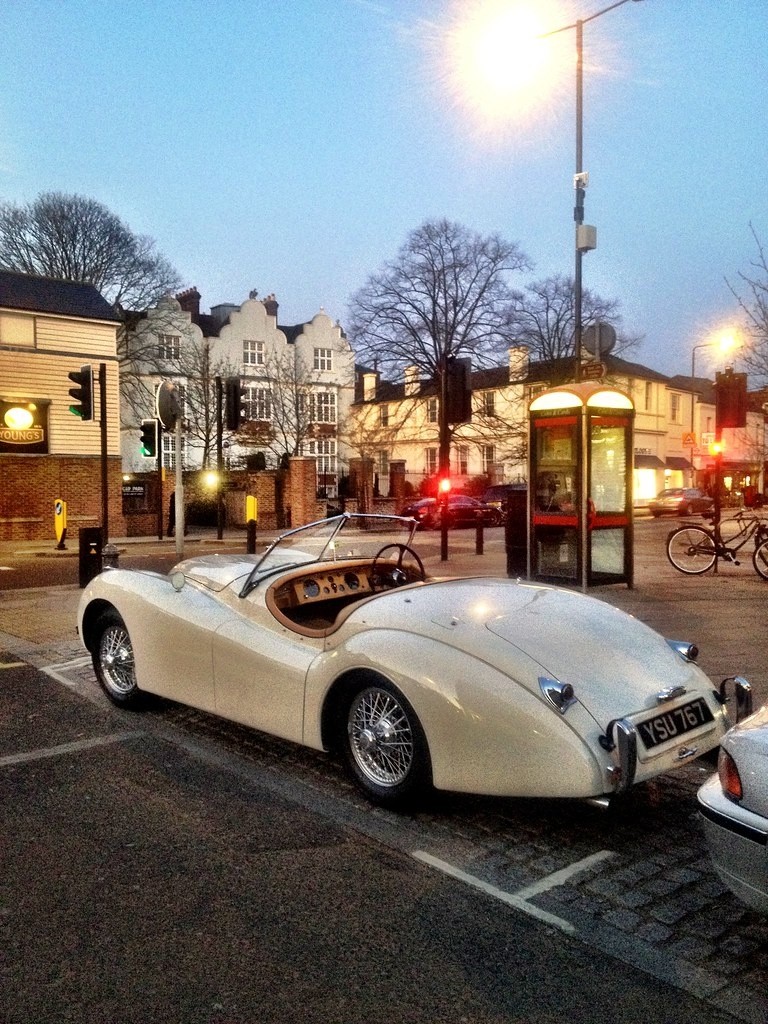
[540,472,568,510]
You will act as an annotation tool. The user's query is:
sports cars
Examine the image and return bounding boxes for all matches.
[74,510,753,811]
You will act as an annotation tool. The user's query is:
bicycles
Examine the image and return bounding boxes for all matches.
[666,495,768,586]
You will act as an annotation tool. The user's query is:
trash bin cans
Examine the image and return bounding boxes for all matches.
[77,526,107,592]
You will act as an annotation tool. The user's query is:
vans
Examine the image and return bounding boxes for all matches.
[481,484,527,522]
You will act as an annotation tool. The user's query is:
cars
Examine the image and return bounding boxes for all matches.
[696,697,768,915]
[649,485,715,516]
[682,326,743,483]
[396,493,501,531]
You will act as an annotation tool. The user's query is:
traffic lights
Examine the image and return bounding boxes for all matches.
[224,376,249,431]
[140,417,159,459]
[66,366,94,422]
[439,478,451,493]
